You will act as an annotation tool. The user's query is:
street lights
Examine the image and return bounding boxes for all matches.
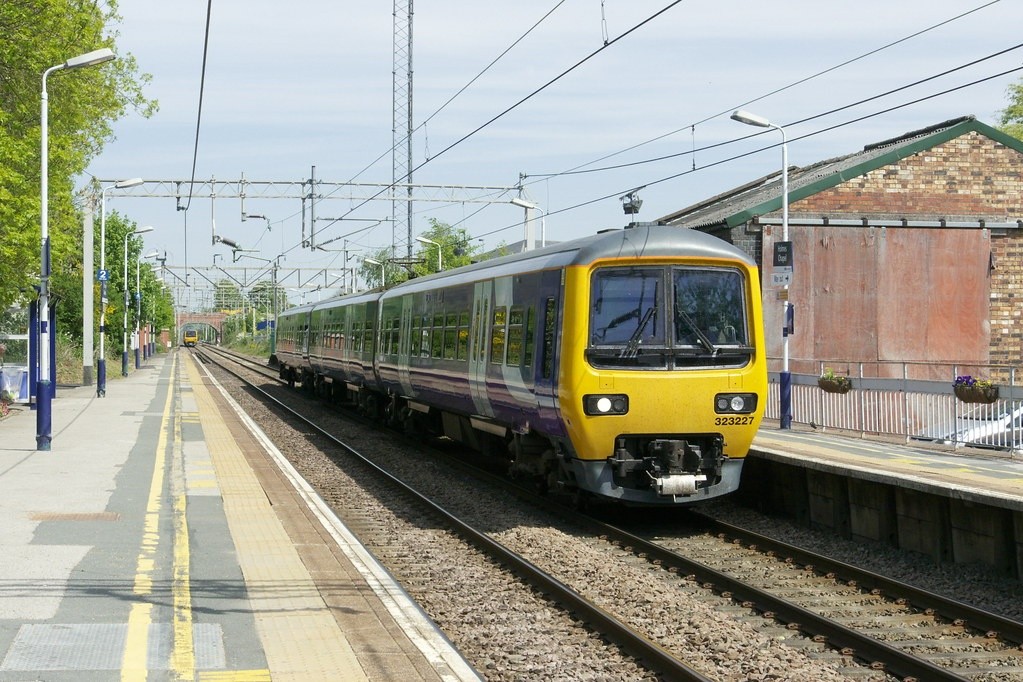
[134,252,160,370]
[364,257,385,286]
[306,283,320,302]
[143,278,168,362]
[121,225,155,377]
[330,273,348,295]
[36,46,119,452]
[96,176,145,396]
[416,237,442,272]
[729,108,792,429]
[289,287,302,304]
[508,198,546,248]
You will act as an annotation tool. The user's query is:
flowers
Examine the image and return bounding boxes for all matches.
[955,375,993,387]
[821,366,848,387]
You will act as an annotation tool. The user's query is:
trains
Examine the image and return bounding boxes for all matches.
[183,331,197,347]
[275,222,768,515]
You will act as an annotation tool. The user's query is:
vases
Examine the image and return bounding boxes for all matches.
[953,385,998,404]
[818,379,851,394]
